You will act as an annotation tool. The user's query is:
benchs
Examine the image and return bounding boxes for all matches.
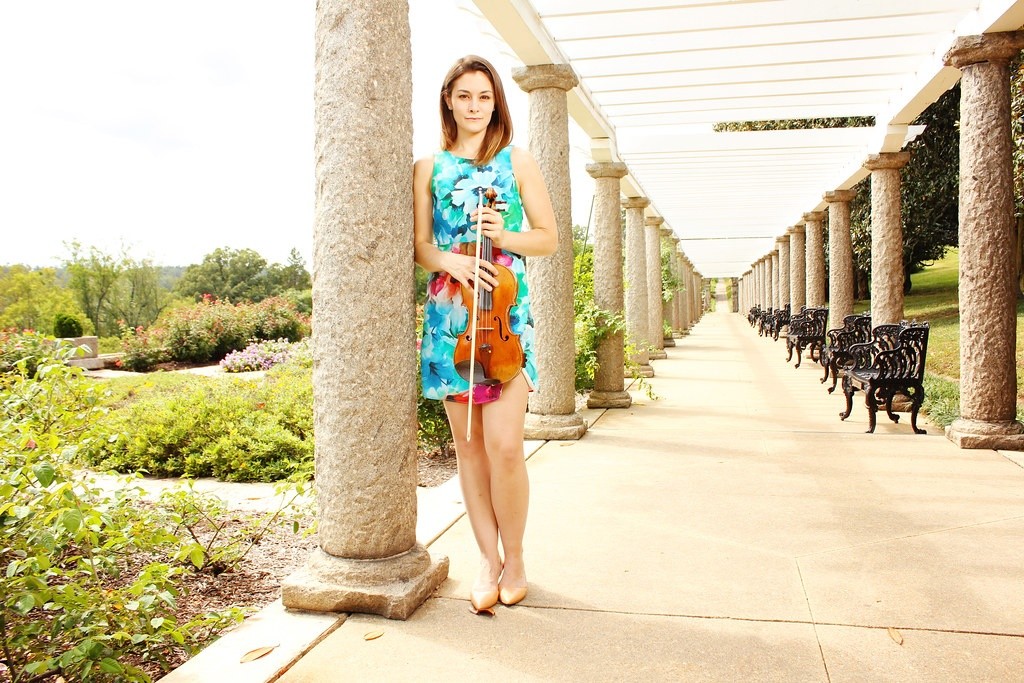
[820,314,873,394]
[785,305,828,368]
[747,301,808,342]
[834,321,929,434]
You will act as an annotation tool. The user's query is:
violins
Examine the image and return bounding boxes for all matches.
[454,187,523,385]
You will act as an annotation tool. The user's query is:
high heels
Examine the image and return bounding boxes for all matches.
[469,555,504,611]
[498,577,528,605]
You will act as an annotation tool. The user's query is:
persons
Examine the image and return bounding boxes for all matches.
[412,54,562,615]
